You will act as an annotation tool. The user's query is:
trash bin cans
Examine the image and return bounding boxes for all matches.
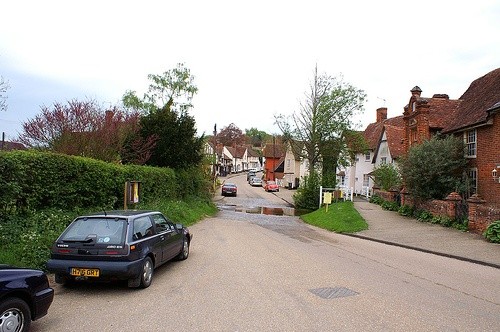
[288,183,293,190]
[124,179,143,205]
[295,178,299,188]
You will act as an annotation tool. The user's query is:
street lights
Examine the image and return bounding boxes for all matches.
[127,175,140,205]
